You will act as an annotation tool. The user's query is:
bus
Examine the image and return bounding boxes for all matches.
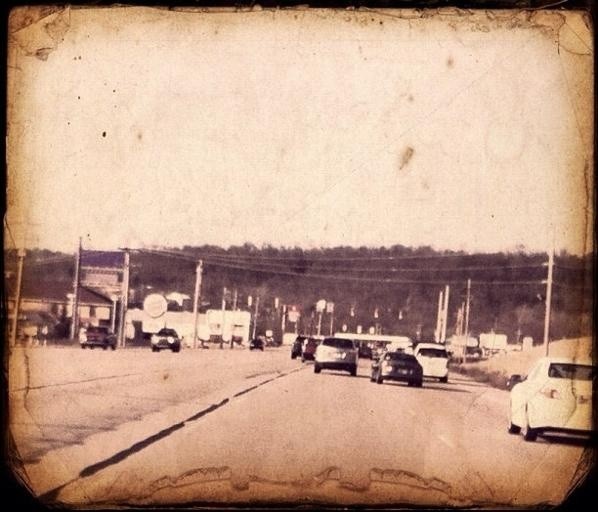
[335,332,409,359]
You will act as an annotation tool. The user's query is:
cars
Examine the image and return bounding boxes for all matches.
[250,340,263,350]
[291,335,356,376]
[80,326,117,350]
[369,343,449,387]
[150,329,184,352]
[506,358,594,440]
